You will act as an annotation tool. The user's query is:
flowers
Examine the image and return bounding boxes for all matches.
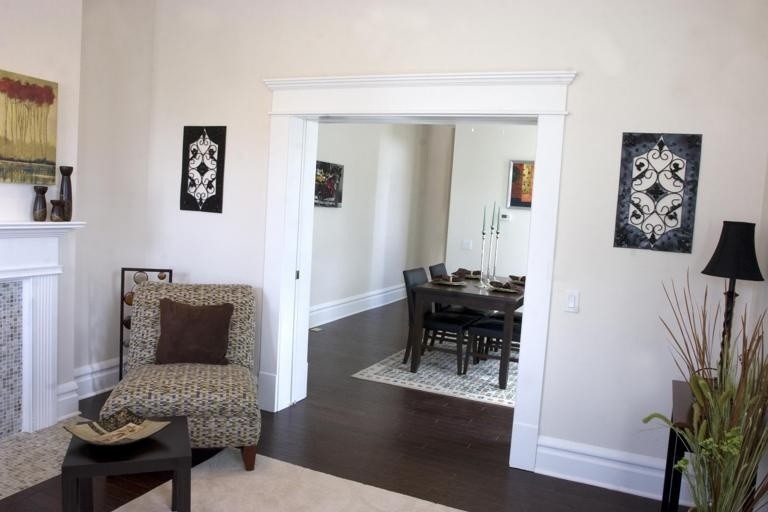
[316,162,340,207]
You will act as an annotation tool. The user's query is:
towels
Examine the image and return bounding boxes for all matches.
[489,280,522,294]
[452,267,480,276]
[433,274,465,283]
[509,275,526,281]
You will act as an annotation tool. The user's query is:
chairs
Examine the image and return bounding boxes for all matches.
[114,281,261,472]
[430,262,492,358]
[402,268,481,375]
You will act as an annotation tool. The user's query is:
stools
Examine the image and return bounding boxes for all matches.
[490,311,522,355]
[464,318,519,375]
[59,417,194,512]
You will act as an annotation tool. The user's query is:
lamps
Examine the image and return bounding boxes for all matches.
[700,221,764,395]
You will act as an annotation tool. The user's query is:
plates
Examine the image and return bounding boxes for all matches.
[62,406,172,446]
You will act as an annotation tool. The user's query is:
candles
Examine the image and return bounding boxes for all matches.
[496,208,501,235]
[491,202,496,233]
[483,207,489,238]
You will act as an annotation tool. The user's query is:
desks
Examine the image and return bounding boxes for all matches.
[662,381,759,512]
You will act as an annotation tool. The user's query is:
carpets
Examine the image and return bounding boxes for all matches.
[351,327,521,409]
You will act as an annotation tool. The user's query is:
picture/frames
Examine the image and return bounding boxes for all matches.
[506,160,534,212]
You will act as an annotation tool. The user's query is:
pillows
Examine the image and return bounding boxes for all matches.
[155,296,233,366]
